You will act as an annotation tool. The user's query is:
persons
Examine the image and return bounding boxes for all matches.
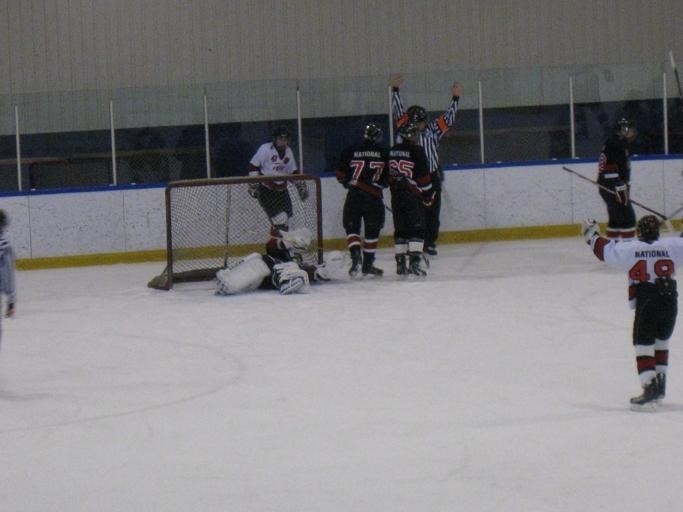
[387,122,435,281]
[248,128,309,239]
[217,228,331,296]
[336,122,390,279]
[596,117,638,243]
[0,209,14,320]
[391,76,460,255]
[579,216,681,404]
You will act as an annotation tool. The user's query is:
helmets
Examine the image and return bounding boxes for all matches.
[406,105,428,123]
[397,120,419,142]
[636,215,660,245]
[364,122,383,142]
[615,116,634,137]
[271,128,290,153]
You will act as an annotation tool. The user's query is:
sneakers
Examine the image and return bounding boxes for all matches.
[408,255,427,276]
[630,384,660,404]
[395,255,413,275]
[423,245,437,255]
[279,277,304,295]
[657,371,666,400]
[348,255,363,276]
[362,256,383,274]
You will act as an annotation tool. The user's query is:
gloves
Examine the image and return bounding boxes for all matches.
[422,187,438,207]
[615,183,630,207]
[247,182,261,198]
[295,180,308,200]
[581,218,601,245]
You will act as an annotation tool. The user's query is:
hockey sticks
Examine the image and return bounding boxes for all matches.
[561,166,676,239]
